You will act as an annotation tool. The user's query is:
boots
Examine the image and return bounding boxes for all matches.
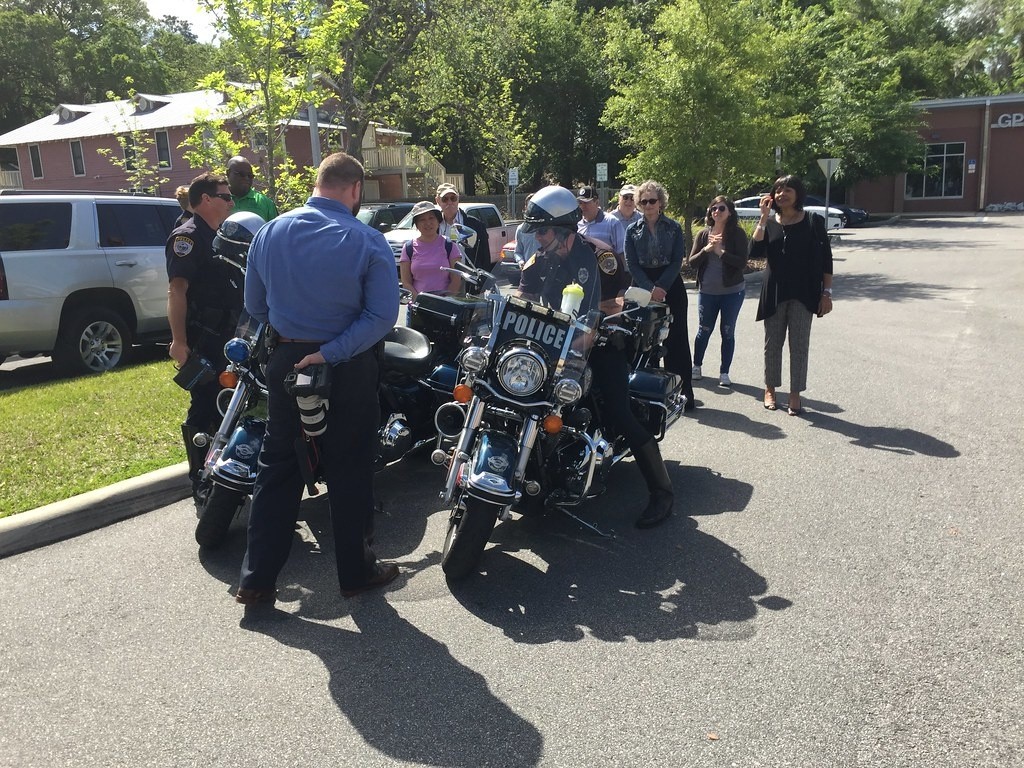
[631,437,674,529]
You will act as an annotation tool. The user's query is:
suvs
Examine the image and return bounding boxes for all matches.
[355,201,414,234]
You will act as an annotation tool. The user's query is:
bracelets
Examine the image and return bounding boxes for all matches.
[757,225,765,230]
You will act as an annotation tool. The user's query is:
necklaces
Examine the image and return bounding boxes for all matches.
[781,213,792,253]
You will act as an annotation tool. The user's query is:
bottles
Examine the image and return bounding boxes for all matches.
[559,283,585,321]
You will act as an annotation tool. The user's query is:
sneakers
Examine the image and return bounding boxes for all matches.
[236,584,276,604]
[341,563,399,598]
[692,366,702,379]
[720,374,731,385]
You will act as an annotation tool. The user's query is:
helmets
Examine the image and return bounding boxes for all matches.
[522,186,583,234]
[212,211,266,258]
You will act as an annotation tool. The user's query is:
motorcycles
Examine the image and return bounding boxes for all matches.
[193,254,496,551]
[430,224,686,579]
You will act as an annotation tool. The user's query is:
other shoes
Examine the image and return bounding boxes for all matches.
[193,485,246,506]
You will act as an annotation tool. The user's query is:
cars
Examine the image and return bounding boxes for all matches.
[804,195,871,226]
[499,239,520,286]
[733,196,849,232]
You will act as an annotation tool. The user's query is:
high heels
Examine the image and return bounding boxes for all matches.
[788,400,801,415]
[764,390,776,409]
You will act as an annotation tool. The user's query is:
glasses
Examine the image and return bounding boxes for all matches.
[539,225,551,235]
[623,196,634,201]
[199,191,232,202]
[711,206,725,212]
[639,199,659,205]
[441,195,457,203]
[229,170,253,178]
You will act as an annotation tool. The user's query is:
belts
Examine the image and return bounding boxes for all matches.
[277,335,324,343]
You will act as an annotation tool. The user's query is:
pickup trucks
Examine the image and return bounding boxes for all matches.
[383,202,525,287]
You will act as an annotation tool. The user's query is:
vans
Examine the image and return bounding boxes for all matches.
[0,187,187,376]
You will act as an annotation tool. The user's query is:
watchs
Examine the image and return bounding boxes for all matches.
[824,288,832,294]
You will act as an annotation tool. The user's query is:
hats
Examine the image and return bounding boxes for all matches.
[410,201,442,227]
[436,183,459,198]
[576,186,598,202]
[620,185,636,196]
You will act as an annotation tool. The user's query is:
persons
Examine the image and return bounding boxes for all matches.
[517,183,672,529]
[577,186,625,265]
[174,185,193,229]
[689,195,749,386]
[399,201,462,327]
[436,183,491,297]
[610,184,644,230]
[748,175,833,416]
[514,194,542,269]
[226,156,279,222]
[623,180,694,412]
[237,153,400,601]
[165,171,235,505]
